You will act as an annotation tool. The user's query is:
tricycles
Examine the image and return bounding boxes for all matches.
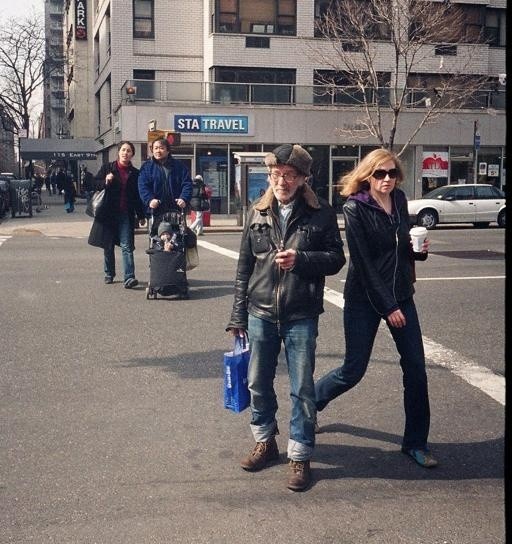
[9,180,33,218]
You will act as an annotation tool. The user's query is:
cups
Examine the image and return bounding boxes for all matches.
[409,226,429,253]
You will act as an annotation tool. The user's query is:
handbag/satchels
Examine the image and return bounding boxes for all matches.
[84,185,111,220]
[180,221,200,271]
[224,328,252,412]
[200,196,210,211]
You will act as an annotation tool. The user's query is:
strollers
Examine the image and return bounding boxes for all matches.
[145,199,190,299]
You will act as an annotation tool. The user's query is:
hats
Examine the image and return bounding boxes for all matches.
[157,222,172,236]
[264,144,314,177]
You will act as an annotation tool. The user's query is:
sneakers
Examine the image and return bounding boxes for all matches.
[400,441,439,469]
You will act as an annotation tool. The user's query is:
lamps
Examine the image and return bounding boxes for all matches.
[126,86,137,102]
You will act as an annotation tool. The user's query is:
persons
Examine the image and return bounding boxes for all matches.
[45,169,76,213]
[225,144,346,491]
[88,141,146,289]
[189,174,210,236]
[313,149,439,468]
[153,221,182,253]
[137,138,193,233]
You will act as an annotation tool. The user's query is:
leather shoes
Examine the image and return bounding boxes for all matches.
[123,277,138,288]
[240,438,279,470]
[104,269,115,284]
[288,460,313,490]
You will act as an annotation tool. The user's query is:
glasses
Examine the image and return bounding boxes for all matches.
[371,167,399,179]
[270,172,298,182]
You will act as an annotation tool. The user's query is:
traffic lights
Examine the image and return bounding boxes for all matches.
[165,132,182,146]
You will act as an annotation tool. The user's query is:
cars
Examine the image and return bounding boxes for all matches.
[0,173,29,191]
[0,181,9,210]
[409,184,506,228]
